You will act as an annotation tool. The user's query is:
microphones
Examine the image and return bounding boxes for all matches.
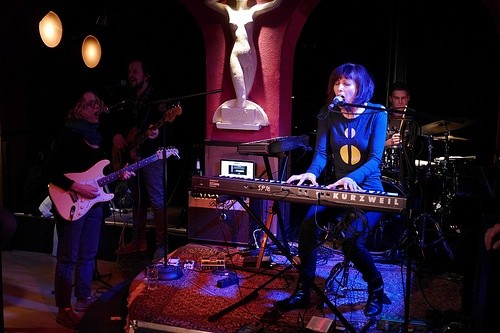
[328,96,343,109]
[104,100,127,114]
[220,213,227,220]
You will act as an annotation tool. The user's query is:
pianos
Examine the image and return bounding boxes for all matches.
[190,173,408,333]
[231,133,311,279]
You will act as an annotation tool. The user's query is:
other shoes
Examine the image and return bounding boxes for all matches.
[116,240,148,254]
[55,307,88,330]
[152,246,168,264]
[74,298,106,313]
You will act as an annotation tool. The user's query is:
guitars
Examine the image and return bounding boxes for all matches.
[47,146,181,221]
[108,103,183,171]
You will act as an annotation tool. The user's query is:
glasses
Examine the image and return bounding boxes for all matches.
[85,100,104,109]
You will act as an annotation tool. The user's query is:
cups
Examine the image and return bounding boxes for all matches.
[147,266,158,290]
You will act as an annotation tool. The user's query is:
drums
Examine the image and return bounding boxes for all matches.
[381,145,402,176]
[363,176,410,256]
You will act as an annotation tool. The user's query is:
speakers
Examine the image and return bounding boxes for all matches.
[188,191,263,247]
[75,278,132,333]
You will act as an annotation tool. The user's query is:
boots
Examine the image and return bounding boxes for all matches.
[362,271,384,316]
[273,276,314,309]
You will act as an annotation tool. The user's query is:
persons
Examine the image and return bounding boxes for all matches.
[107,58,167,254]
[205,0,281,108]
[277,63,387,316]
[484,223,500,251]
[50,91,137,329]
[385,82,421,153]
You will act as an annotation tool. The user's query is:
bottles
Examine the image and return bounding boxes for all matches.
[191,158,202,197]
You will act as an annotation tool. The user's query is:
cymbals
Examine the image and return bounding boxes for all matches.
[433,135,468,141]
[419,120,466,134]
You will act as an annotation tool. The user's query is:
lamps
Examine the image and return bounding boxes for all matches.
[39,10,63,48]
[82,35,102,69]
